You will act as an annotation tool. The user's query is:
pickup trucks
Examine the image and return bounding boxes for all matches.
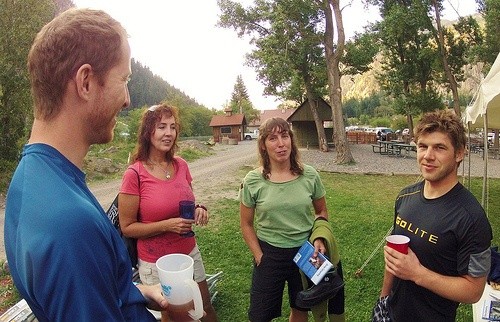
[243,129,260,141]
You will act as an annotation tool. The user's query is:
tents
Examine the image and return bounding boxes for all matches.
[458,51,500,219]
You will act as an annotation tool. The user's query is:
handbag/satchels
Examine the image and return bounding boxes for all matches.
[105,194,138,270]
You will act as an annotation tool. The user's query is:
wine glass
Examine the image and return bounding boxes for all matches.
[179,200,195,238]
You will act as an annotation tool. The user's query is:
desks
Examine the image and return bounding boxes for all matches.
[391,144,412,158]
[378,141,393,155]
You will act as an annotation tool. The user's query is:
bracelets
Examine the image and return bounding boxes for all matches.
[194,204,208,211]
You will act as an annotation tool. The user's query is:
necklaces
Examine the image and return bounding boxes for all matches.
[146,156,172,180]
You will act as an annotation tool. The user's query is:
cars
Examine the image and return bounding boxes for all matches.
[485,132,500,144]
[376,128,398,141]
[402,128,411,136]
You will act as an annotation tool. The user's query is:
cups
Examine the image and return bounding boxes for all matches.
[385,235,410,255]
[155,254,203,322]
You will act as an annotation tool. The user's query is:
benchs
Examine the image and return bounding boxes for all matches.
[372,140,416,158]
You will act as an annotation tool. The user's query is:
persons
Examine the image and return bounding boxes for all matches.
[368,109,494,322]
[115,102,220,322]
[2,5,207,322]
[235,116,330,322]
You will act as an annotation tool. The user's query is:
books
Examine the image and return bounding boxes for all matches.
[292,241,333,285]
